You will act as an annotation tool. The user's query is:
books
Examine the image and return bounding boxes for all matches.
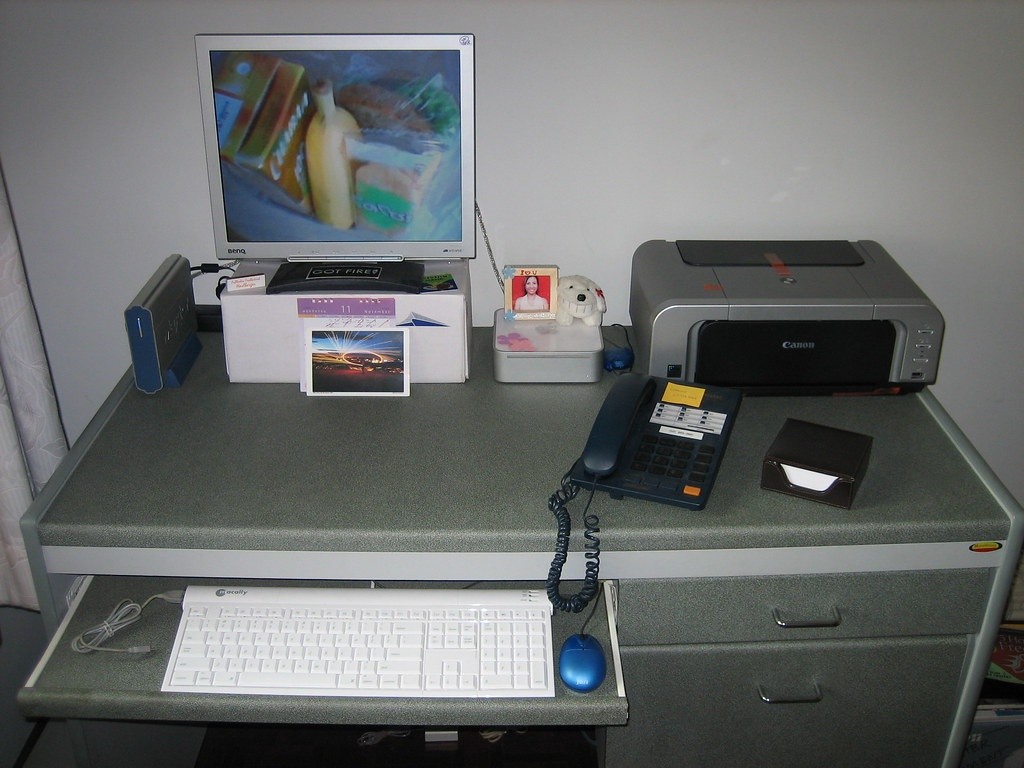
[421,273,458,293]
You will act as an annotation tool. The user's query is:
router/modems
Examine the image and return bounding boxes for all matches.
[125,252,197,395]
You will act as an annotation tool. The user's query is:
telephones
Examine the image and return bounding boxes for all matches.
[572,373,743,511]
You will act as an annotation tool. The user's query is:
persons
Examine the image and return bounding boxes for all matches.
[514,276,549,311]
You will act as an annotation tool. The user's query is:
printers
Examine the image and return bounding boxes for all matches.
[629,239,947,398]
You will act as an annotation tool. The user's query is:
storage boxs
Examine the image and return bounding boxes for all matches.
[494,308,604,384]
[761,418,873,511]
[220,258,471,385]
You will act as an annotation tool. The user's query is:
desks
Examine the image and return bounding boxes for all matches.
[14,328,1024,768]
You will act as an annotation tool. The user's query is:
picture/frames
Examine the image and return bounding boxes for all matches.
[307,327,410,397]
[504,264,559,320]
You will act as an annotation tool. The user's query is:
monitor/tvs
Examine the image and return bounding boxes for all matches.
[194,34,475,290]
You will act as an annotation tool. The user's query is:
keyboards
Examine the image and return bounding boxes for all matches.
[160,586,555,700]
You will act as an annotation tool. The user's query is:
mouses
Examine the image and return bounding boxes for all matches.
[558,634,605,694]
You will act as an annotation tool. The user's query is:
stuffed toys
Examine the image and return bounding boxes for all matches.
[556,274,606,327]
